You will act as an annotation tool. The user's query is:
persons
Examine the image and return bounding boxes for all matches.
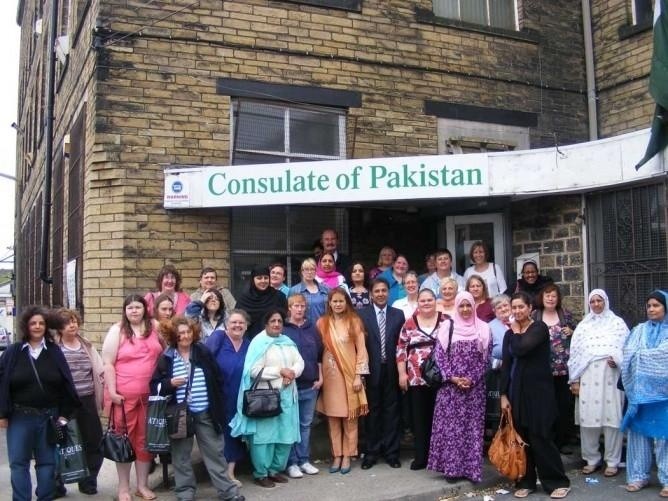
[426,242,577,498]
[570,290,631,478]
[622,289,668,500]
[290,230,467,469]
[1,307,103,500]
[100,264,323,501]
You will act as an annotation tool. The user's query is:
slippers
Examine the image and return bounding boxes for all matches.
[551,486,571,499]
[513,488,534,498]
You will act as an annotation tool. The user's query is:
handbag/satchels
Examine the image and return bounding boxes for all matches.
[487,420,527,482]
[45,415,64,445]
[145,394,174,455]
[242,388,283,418]
[55,418,92,485]
[420,358,443,390]
[165,400,195,441]
[94,430,136,464]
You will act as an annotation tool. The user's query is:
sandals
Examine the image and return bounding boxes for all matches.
[582,465,598,474]
[626,480,648,492]
[603,466,618,477]
[116,487,134,501]
[135,486,158,500]
[659,487,668,497]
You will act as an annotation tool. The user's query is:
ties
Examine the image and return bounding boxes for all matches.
[378,310,387,361]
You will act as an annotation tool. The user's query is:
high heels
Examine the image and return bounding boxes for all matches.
[340,459,352,474]
[329,460,340,473]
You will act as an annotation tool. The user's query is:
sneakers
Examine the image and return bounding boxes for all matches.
[299,462,319,475]
[408,459,428,470]
[80,486,100,496]
[54,490,67,498]
[287,465,304,478]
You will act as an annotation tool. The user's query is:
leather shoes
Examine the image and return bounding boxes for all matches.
[254,477,276,488]
[361,454,378,469]
[268,474,288,484]
[386,456,402,468]
